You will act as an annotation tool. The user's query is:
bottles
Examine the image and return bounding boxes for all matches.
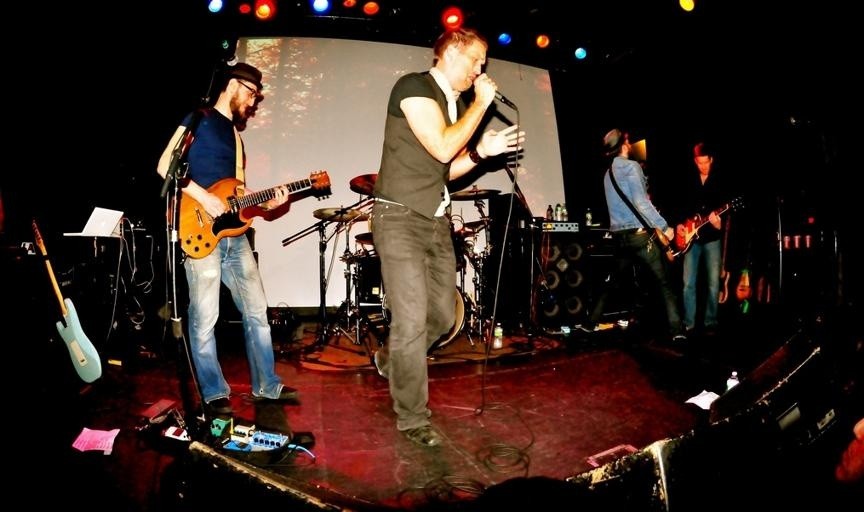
[561,203,568,221]
[726,371,738,390]
[547,205,553,221]
[493,323,502,349]
[555,204,561,221]
[586,208,592,226]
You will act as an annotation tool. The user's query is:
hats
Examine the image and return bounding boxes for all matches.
[225,63,264,89]
[603,129,625,157]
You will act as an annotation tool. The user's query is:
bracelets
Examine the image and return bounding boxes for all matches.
[468,149,487,166]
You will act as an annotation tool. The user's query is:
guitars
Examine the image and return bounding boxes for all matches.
[26,219,103,383]
[666,196,746,260]
[718,213,733,304]
[166,171,333,259]
[652,226,678,261]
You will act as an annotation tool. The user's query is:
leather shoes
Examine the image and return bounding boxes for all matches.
[373,349,391,381]
[398,425,440,446]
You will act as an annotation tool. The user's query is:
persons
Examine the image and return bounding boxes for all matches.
[155,63,299,416]
[599,129,691,346]
[830,414,864,482]
[373,26,526,447]
[672,142,725,337]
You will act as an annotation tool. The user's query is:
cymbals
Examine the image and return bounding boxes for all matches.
[310,206,372,224]
[348,172,380,197]
[444,185,503,201]
[352,230,378,246]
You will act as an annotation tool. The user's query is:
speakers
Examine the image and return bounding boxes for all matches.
[564,228,593,330]
[488,215,564,333]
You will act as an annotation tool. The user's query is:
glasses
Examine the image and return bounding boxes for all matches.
[237,80,263,102]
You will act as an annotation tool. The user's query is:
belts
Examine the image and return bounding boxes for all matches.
[611,227,639,235]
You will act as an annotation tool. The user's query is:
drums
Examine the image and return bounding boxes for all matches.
[310,171,503,351]
[381,281,466,348]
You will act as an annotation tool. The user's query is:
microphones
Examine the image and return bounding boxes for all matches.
[472,79,518,111]
[218,33,238,67]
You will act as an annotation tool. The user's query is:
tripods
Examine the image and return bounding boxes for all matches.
[460,268,483,348]
[301,227,340,352]
[328,227,377,346]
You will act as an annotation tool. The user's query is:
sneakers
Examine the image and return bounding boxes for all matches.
[206,397,232,414]
[253,385,298,401]
